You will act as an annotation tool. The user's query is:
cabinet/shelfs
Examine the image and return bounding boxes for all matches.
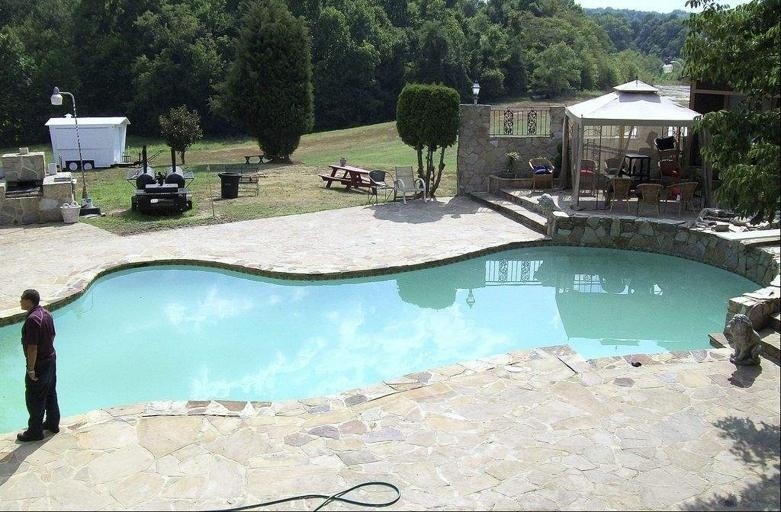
[620,152,650,196]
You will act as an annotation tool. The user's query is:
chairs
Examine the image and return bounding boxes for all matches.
[602,159,699,219]
[579,159,596,197]
[368,166,428,207]
[528,156,556,193]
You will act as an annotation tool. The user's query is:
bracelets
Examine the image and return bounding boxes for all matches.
[26,368,35,374]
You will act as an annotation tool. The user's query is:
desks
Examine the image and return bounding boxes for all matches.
[326,164,370,192]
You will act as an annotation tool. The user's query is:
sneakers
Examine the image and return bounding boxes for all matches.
[17,430,45,442]
[42,421,60,434]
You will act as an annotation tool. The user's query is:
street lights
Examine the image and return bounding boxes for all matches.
[50,87,88,199]
[470,81,482,105]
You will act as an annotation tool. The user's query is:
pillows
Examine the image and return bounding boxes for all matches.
[533,164,551,176]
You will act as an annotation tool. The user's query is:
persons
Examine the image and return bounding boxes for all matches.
[16,288,61,441]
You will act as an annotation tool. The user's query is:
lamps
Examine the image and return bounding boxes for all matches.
[470,77,482,105]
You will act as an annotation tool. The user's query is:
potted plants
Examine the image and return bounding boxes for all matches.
[500,148,521,178]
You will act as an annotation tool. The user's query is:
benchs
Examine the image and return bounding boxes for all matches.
[357,176,370,190]
[223,167,261,197]
[318,174,353,192]
